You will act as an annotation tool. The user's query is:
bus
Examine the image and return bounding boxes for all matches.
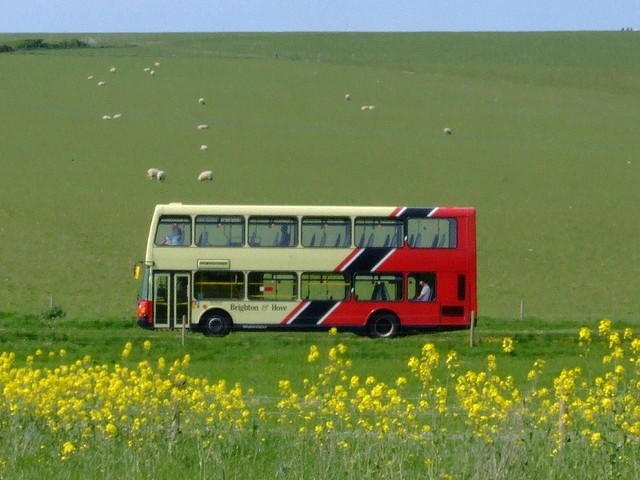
[134,201,481,339]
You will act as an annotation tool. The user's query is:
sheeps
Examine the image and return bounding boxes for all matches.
[148,168,159,179]
[198,170,213,181]
[157,171,167,183]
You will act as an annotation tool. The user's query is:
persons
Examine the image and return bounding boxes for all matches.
[164,223,181,246]
[279,224,290,245]
[415,278,430,301]
